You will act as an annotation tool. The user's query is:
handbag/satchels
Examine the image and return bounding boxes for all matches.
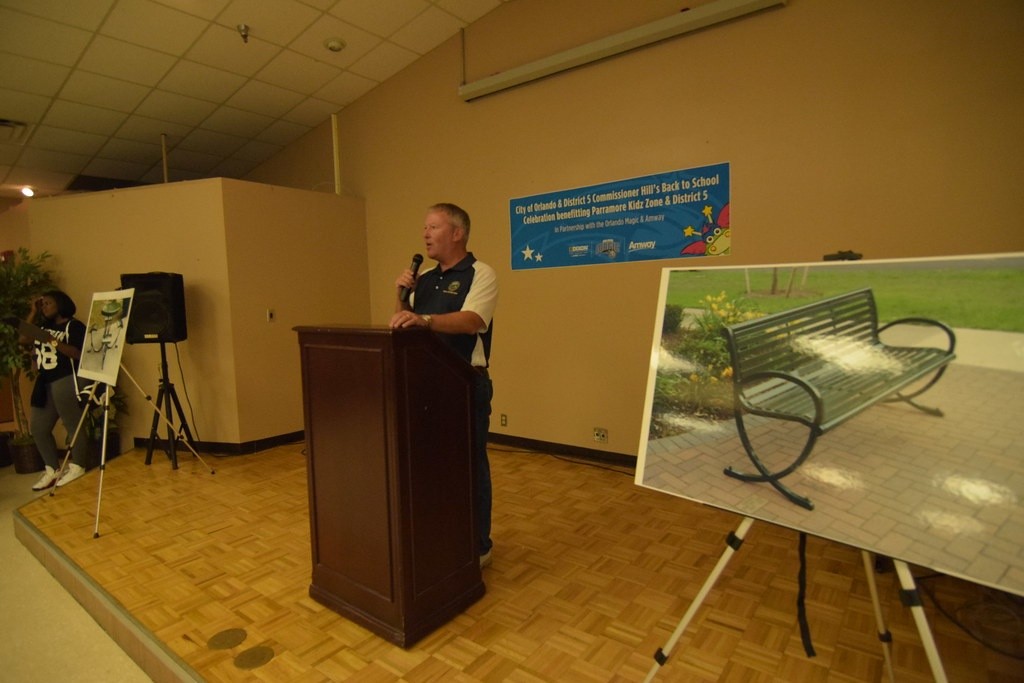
[77,380,115,411]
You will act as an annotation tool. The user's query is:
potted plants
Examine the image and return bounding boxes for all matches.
[0,244,57,475]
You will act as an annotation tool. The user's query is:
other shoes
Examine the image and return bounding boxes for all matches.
[479,550,492,570]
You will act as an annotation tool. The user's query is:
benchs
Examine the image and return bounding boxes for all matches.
[718,287,958,512]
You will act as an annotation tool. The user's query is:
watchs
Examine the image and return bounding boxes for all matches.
[422,315,431,328]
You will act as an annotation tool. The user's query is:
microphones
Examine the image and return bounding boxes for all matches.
[400,253,423,302]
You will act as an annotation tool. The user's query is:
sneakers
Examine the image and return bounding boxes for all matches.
[32,465,61,490]
[57,463,85,487]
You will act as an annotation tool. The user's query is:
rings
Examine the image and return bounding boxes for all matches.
[406,314,411,319]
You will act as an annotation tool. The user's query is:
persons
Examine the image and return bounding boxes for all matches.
[389,203,496,570]
[19,291,90,491]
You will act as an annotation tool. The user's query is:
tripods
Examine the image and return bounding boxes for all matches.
[145,343,200,470]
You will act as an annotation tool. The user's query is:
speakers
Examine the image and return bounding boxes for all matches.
[120,271,187,345]
